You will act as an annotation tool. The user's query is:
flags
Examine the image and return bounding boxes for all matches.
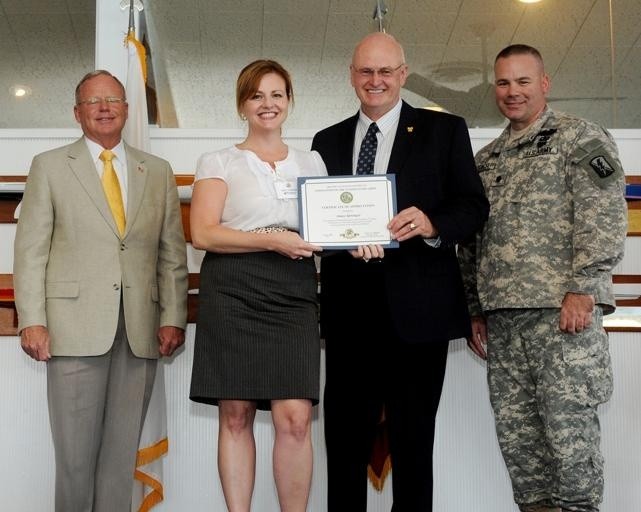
[122,30,170,510]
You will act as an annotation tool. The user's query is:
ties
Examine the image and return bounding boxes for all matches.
[98,149,126,237]
[356,121,381,176]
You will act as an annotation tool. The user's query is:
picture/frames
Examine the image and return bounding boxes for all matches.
[297,173,400,250]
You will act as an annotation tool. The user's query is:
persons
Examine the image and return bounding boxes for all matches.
[186,58,386,509]
[308,32,492,509]
[456,44,632,510]
[13,69,191,509]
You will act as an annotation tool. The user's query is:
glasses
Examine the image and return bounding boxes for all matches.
[77,96,126,106]
[351,63,403,77]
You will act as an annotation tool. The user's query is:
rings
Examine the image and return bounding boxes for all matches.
[363,257,370,263]
[409,223,417,230]
